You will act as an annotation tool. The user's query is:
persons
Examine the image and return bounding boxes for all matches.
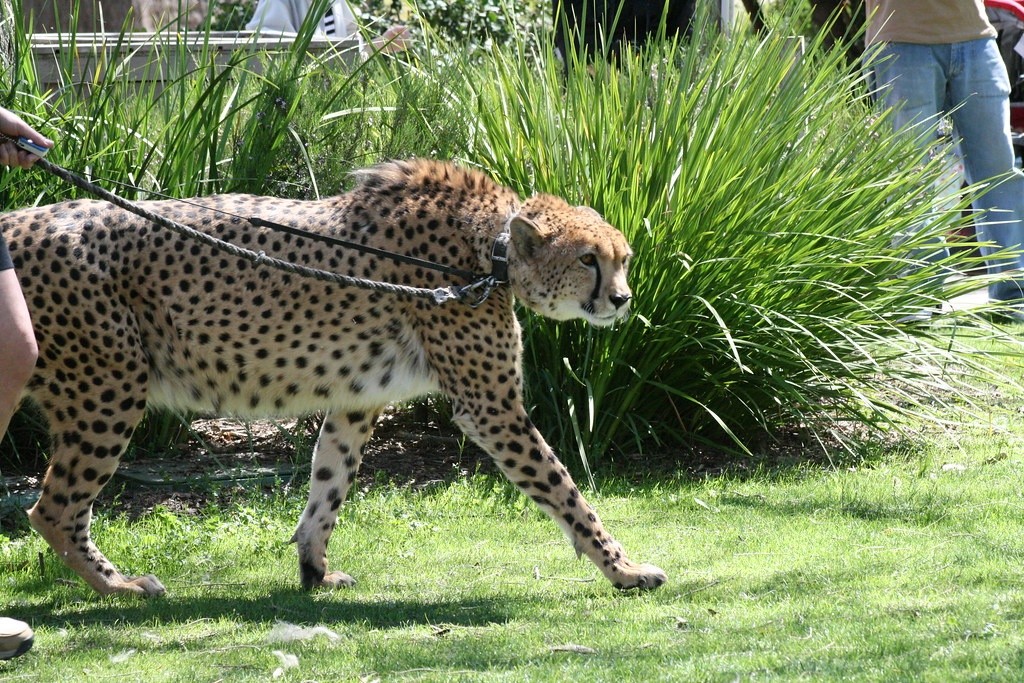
[245,0,413,57]
[0,106,56,660]
[552,0,1024,313]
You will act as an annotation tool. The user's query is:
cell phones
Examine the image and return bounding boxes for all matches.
[17,136,49,157]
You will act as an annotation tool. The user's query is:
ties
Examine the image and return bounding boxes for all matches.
[324,3,336,37]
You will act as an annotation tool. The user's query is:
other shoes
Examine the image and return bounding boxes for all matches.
[896,310,933,323]
[0,617,34,660]
[1011,308,1024,321]
